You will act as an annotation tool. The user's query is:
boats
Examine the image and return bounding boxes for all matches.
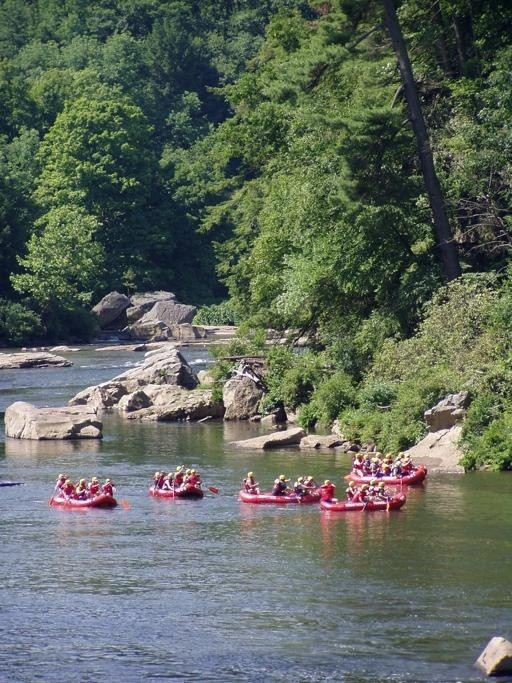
[48,493,117,508]
[238,466,427,512]
[148,483,203,498]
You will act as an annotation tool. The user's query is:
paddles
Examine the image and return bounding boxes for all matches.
[199,484,219,494]
[50,488,59,504]
[290,481,302,505]
[386,491,390,512]
[115,490,129,508]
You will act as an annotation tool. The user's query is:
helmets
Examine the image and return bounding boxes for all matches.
[58,474,111,485]
[324,479,331,486]
[347,478,386,497]
[247,472,254,478]
[156,466,196,478]
[308,476,313,481]
[298,477,303,482]
[354,451,411,471]
[280,474,286,481]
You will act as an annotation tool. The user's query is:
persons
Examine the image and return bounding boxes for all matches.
[242,470,391,506]
[54,472,116,501]
[151,466,202,492]
[352,452,414,478]
